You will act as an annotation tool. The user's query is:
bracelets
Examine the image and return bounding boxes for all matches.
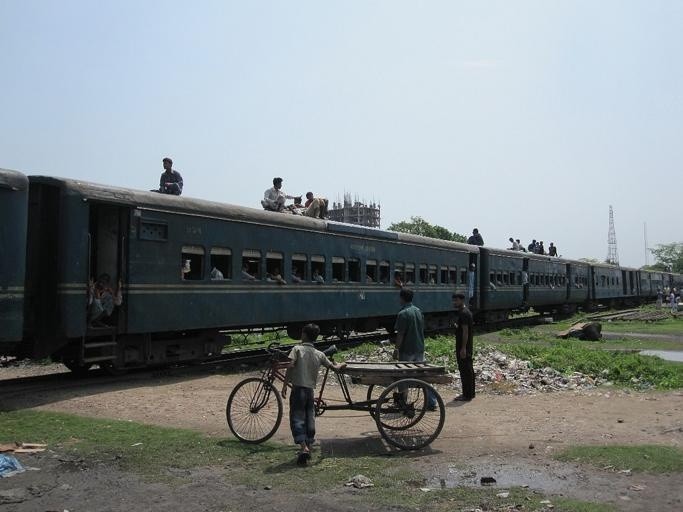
[394,348,399,352]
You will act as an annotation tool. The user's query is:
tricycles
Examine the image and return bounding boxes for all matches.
[226,342,453,450]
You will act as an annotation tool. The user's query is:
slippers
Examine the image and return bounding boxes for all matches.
[297,448,313,465]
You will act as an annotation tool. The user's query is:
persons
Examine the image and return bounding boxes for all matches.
[311,267,323,284]
[210,264,224,280]
[515,239,527,253]
[449,292,476,402]
[280,323,348,467]
[266,265,287,284]
[259,177,303,213]
[392,287,438,413]
[292,265,302,282]
[505,237,518,251]
[285,196,326,220]
[88,271,122,329]
[151,156,183,196]
[305,192,328,220]
[534,242,539,253]
[241,263,256,280]
[466,227,484,246]
[466,262,476,307]
[538,241,544,254]
[521,268,529,304]
[527,239,535,252]
[655,285,680,319]
[548,242,557,257]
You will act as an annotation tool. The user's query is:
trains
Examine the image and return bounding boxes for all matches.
[0,167,683,378]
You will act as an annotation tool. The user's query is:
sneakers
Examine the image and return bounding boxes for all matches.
[387,395,473,411]
